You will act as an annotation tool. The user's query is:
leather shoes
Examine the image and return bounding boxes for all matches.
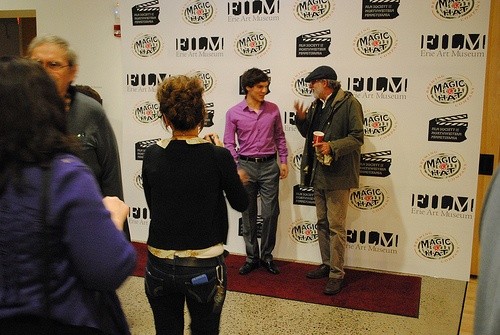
[305,263,331,279]
[323,277,347,296]
[259,259,279,275]
[238,261,260,276]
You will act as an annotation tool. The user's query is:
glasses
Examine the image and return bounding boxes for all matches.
[309,80,319,85]
[33,58,70,71]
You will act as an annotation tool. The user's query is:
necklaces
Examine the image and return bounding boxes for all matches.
[173,135,198,137]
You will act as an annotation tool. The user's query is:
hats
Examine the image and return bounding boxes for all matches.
[305,65,338,82]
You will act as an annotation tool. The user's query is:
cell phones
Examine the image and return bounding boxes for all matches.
[191,274,208,285]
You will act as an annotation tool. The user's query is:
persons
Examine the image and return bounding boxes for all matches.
[473,164,500,335]
[0,53,137,335]
[141,75,249,335]
[294,65,364,295]
[24,34,131,243]
[224,68,288,275]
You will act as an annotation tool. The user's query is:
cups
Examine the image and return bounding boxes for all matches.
[313,131,324,145]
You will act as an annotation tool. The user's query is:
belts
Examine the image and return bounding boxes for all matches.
[240,155,277,164]
[147,248,230,268]
